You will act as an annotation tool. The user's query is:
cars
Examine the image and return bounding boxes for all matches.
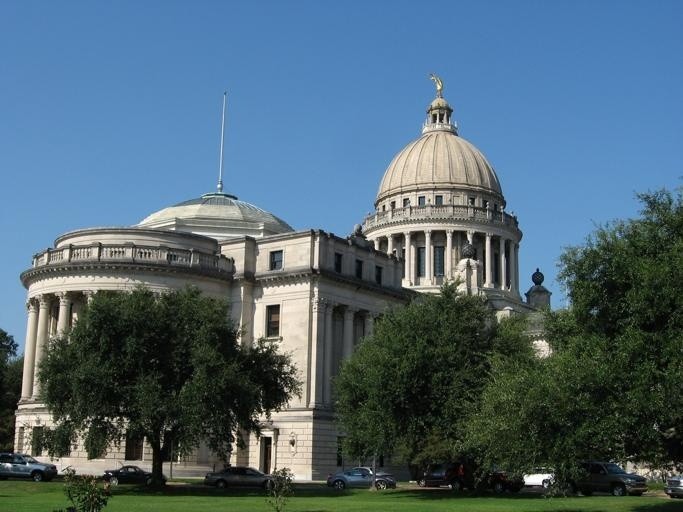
[353,466,392,475]
[202,466,285,491]
[414,465,447,487]
[521,466,556,488]
[663,472,683,499]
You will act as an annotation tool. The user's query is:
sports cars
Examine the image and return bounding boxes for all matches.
[103,465,168,486]
[324,469,396,491]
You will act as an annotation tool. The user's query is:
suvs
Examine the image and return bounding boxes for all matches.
[445,462,524,495]
[574,460,646,497]
[0,451,57,483]
